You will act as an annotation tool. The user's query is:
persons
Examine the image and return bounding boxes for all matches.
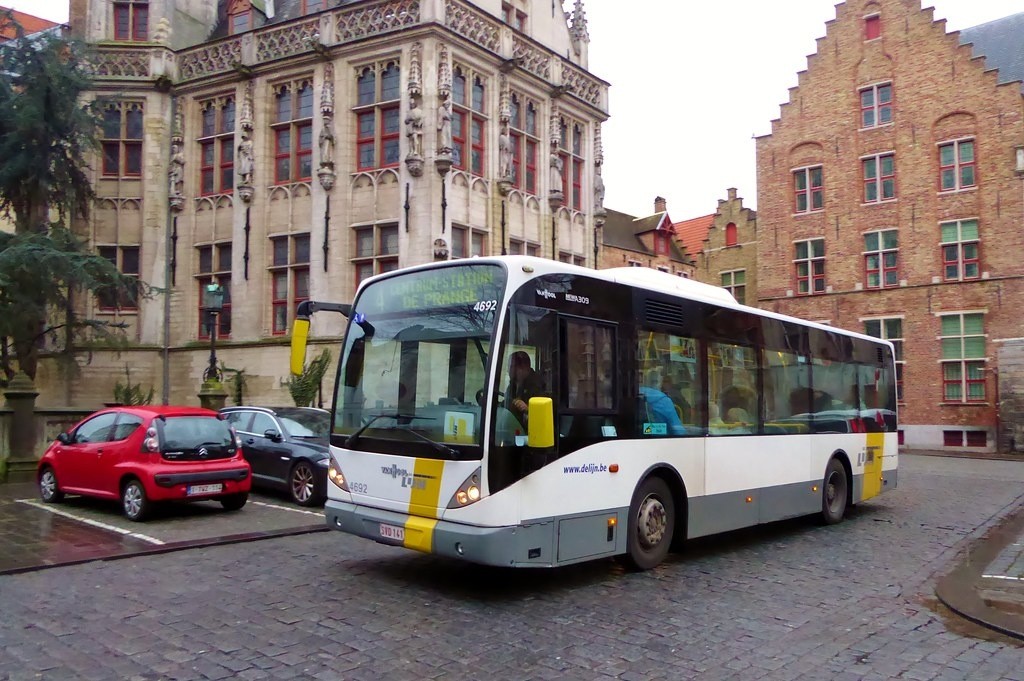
[501,351,546,436]
[638,367,833,435]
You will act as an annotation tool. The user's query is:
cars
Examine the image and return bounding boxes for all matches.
[36,404,253,523]
[218,405,333,508]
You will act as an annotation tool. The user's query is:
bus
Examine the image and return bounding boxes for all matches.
[290,253,899,572]
